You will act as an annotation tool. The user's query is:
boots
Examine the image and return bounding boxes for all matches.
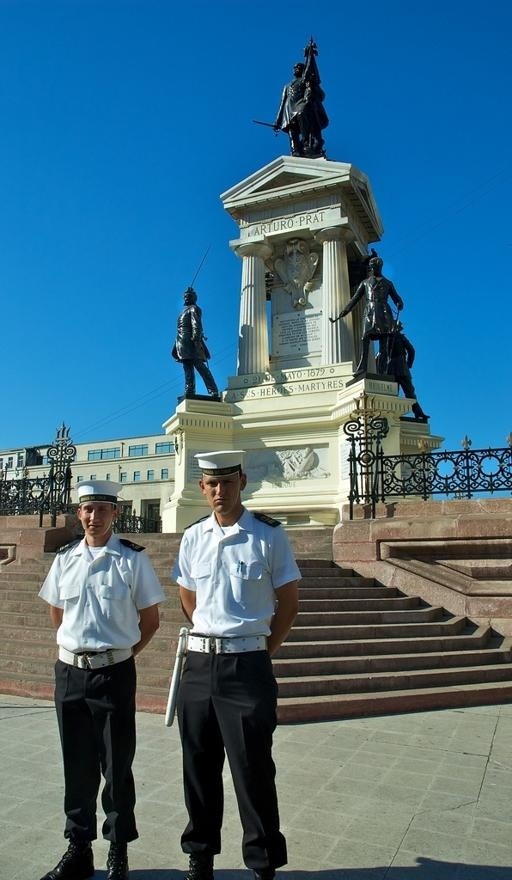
[183,854,213,879]
[41,841,94,880]
[107,843,129,880]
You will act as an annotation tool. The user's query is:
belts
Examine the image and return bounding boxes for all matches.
[58,647,133,668]
[185,635,268,654]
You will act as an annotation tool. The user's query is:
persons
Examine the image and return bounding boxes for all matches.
[272,61,310,156]
[336,256,405,376]
[38,480,166,878]
[172,285,220,398]
[385,318,434,417]
[173,446,303,880]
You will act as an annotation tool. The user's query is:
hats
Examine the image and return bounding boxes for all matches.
[73,480,122,506]
[194,450,247,477]
